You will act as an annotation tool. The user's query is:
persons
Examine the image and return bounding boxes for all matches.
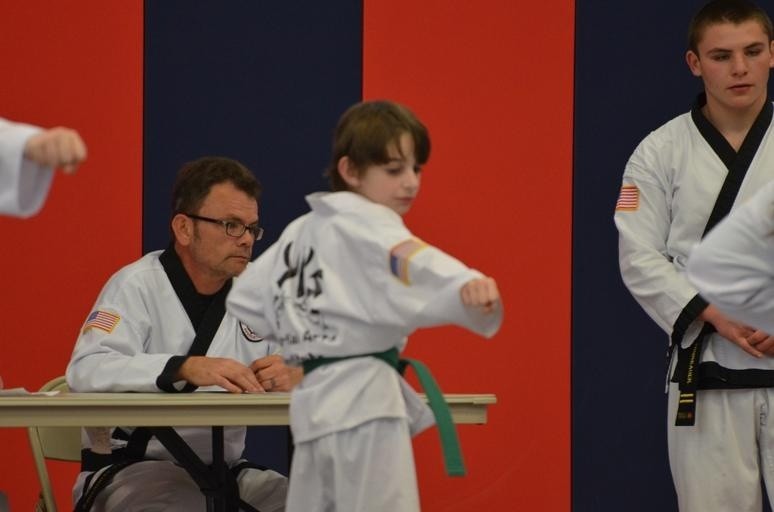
[225,101,500,511]
[614,1,773,512]
[684,179,772,337]
[0,120,87,218]
[64,156,305,510]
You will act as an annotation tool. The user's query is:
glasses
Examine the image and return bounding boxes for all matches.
[185,211,265,242]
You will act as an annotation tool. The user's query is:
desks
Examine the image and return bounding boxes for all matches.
[0,388,498,511]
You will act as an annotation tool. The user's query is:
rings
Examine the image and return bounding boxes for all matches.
[269,378,277,386]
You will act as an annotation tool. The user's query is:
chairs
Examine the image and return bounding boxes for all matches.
[26,372,89,512]
